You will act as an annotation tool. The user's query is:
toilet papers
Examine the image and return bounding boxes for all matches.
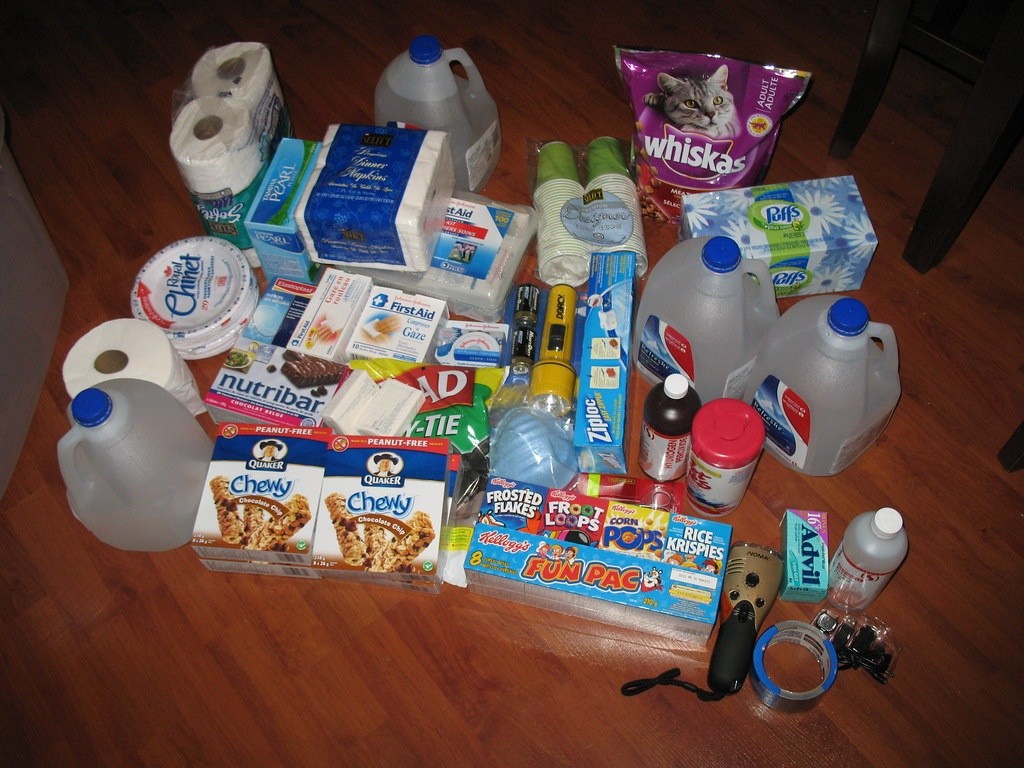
[191,42,297,158]
[62,319,207,418]
[170,97,274,268]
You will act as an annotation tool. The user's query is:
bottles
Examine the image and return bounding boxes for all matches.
[57,378,216,553]
[825,507,908,613]
[741,295,901,476]
[632,236,780,407]
[637,373,703,481]
[373,35,502,195]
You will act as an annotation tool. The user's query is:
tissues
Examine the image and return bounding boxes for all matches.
[294,124,456,272]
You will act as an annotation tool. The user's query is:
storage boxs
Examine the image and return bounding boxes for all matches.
[190,137,878,645]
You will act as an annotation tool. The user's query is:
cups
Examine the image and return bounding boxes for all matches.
[532,136,649,287]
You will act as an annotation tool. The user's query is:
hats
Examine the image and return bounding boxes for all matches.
[374,454,399,466]
[260,441,283,451]
